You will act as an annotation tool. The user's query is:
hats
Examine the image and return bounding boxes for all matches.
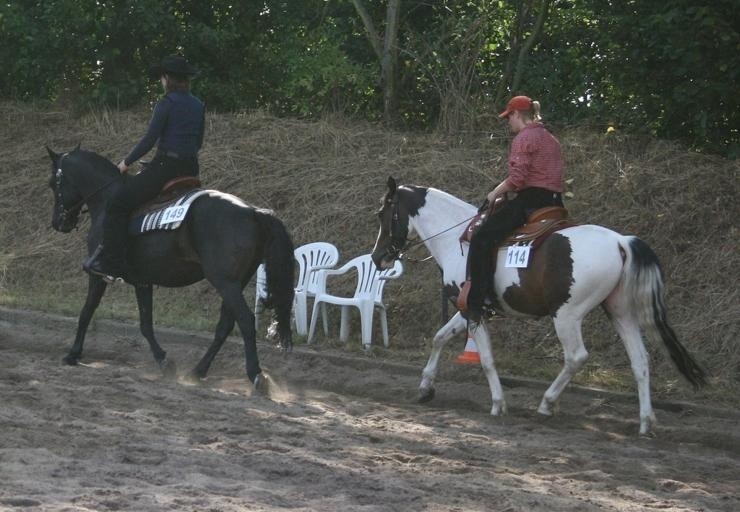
[499,94,532,118]
[150,55,197,79]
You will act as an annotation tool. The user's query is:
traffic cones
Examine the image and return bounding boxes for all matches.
[454,333,481,364]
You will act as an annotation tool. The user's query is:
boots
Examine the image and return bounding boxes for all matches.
[83,216,129,285]
[450,254,489,321]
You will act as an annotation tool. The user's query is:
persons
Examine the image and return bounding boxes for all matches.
[460,94,563,316]
[82,56,206,279]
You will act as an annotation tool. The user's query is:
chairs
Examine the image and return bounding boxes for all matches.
[307,253,403,349]
[253,241,339,337]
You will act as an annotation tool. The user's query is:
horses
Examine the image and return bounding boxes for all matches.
[45,141,295,393]
[372,175,708,442]
